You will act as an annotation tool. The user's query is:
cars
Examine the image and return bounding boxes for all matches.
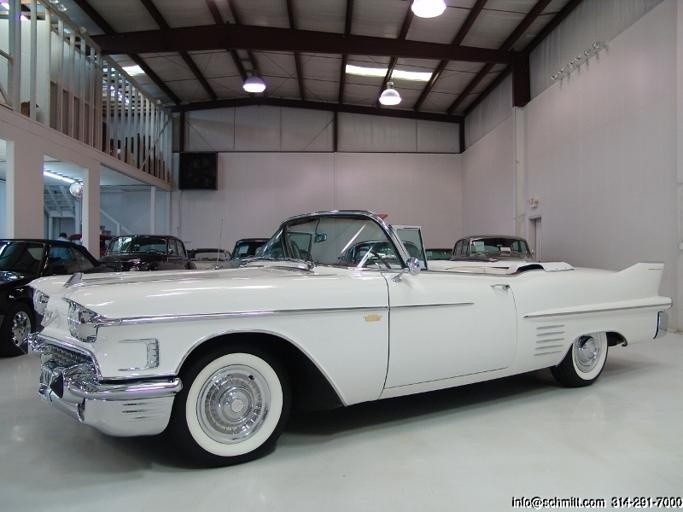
[419,244,452,259]
[0,235,118,357]
[188,244,230,271]
[99,229,197,273]
[57,230,119,269]
[230,236,303,269]
[451,232,538,263]
[338,239,425,272]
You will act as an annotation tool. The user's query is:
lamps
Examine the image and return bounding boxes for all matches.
[42,171,79,184]
[410,0,449,20]
[379,55,402,106]
[241,46,267,93]
[548,39,604,81]
[1,1,27,21]
[107,84,131,106]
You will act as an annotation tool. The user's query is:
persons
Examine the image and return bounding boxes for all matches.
[48,232,81,262]
[102,230,111,249]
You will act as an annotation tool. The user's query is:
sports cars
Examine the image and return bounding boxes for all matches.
[25,207,673,465]
